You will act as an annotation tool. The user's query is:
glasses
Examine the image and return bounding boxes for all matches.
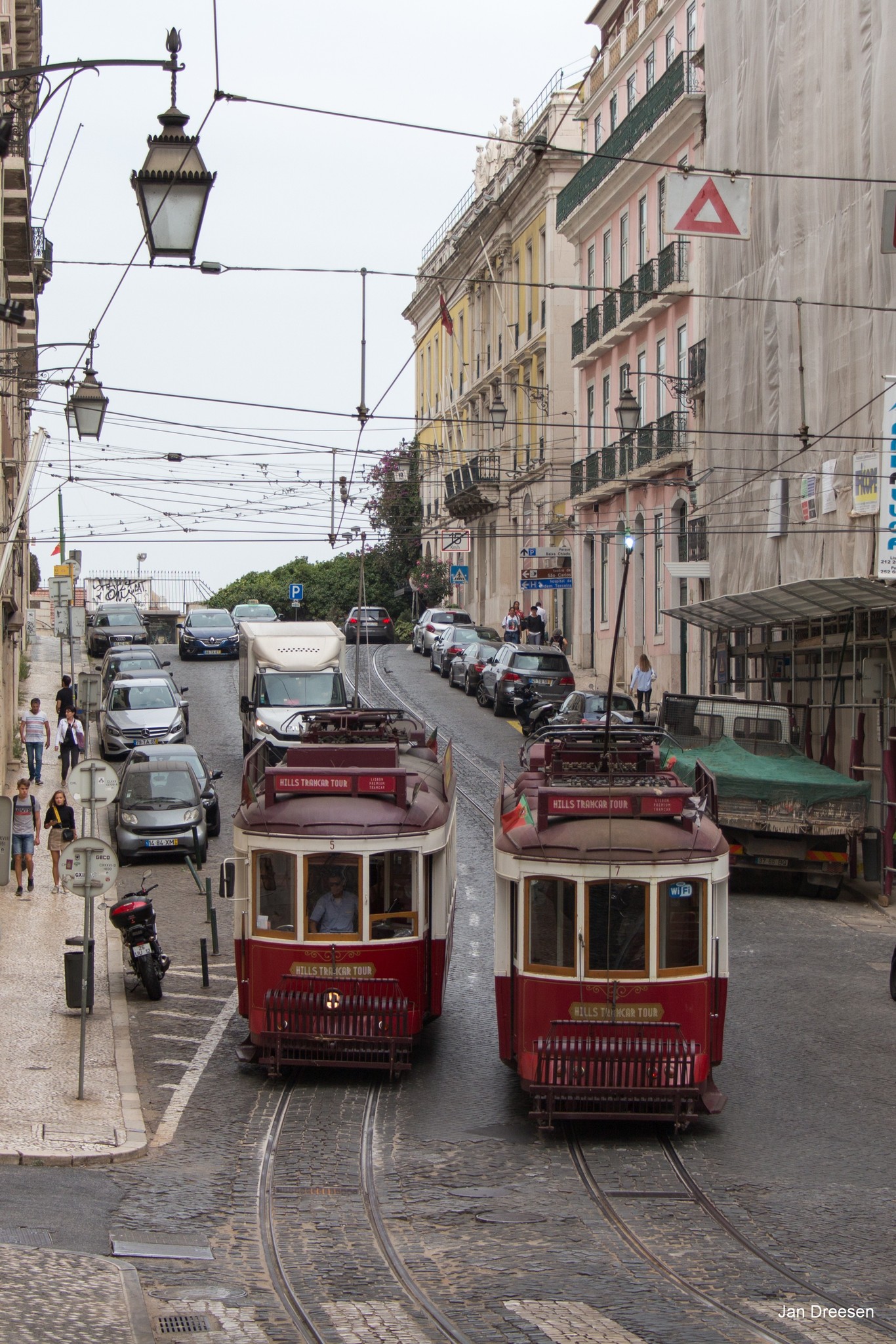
[330,882,343,887]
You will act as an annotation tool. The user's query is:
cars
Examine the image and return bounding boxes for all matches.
[95,644,189,760]
[548,689,658,758]
[231,599,284,633]
[84,602,150,658]
[338,607,395,645]
[116,744,224,869]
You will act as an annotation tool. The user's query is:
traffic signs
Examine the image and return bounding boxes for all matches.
[519,547,571,557]
[520,569,573,590]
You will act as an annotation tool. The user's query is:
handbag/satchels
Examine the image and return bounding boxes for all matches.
[76,732,84,752]
[63,828,74,840]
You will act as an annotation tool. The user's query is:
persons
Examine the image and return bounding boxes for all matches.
[550,628,568,654]
[529,602,548,645]
[70,672,94,755]
[526,606,542,645]
[55,705,84,786]
[630,654,654,712]
[19,698,51,785]
[502,607,522,644]
[56,675,77,759]
[12,778,41,896]
[309,871,359,934]
[507,601,524,644]
[44,790,77,894]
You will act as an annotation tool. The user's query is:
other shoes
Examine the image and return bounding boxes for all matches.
[35,780,44,785]
[28,777,34,781]
[15,886,22,895]
[62,882,69,893]
[27,876,34,891]
[62,779,66,786]
[52,886,59,894]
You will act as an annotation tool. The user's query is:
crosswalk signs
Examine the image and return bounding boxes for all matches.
[450,566,469,584]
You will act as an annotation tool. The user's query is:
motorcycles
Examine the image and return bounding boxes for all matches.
[98,869,172,1002]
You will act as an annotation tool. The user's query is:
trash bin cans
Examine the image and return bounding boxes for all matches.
[64,936,95,1009]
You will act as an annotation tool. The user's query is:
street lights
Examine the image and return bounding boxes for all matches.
[138,553,146,577]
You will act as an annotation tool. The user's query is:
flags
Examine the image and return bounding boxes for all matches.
[440,295,453,336]
[51,541,61,555]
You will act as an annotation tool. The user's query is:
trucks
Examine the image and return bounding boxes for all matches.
[653,690,870,900]
[238,621,360,775]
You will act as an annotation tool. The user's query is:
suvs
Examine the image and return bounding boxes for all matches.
[476,641,575,717]
[412,608,507,696]
[177,608,239,661]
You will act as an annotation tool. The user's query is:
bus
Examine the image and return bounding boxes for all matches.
[220,536,457,1091]
[494,537,730,1142]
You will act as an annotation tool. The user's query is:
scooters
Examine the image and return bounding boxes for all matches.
[505,676,553,740]
